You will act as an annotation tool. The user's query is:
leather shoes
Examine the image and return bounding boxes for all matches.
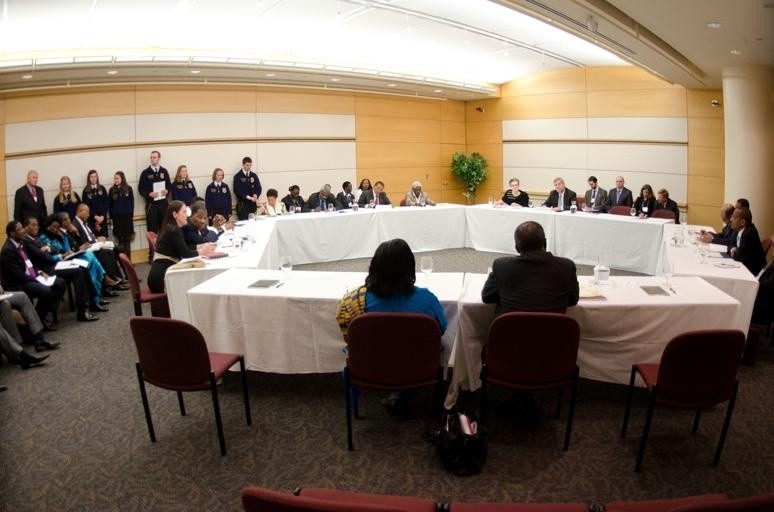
[34,339,61,352]
[76,311,100,322]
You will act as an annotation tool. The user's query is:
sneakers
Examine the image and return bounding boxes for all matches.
[112,285,130,291]
[102,290,120,297]
[42,325,58,333]
[102,278,123,288]
[89,304,110,312]
[96,299,113,306]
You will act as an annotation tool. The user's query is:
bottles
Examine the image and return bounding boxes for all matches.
[570,201,576,213]
[593,253,610,286]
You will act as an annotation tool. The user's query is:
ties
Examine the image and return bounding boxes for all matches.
[18,246,37,281]
[246,173,249,176]
[375,195,379,205]
[320,199,325,211]
[217,184,220,189]
[617,190,621,201]
[84,222,96,242]
[592,190,596,199]
[558,192,563,207]
[154,167,158,172]
[30,186,38,202]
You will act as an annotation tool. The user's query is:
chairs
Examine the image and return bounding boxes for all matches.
[754,257,774,284]
[145,227,160,260]
[128,309,250,460]
[468,308,586,456]
[240,486,774,511]
[328,315,450,460]
[114,249,170,318]
[759,238,774,257]
[616,323,746,468]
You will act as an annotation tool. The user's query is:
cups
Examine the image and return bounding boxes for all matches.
[280,255,292,280]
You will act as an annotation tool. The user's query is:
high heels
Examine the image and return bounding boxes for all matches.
[17,349,50,371]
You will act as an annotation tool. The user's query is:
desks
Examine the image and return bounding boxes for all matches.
[164,203,758,390]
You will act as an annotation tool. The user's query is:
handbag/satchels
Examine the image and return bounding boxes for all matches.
[433,407,489,476]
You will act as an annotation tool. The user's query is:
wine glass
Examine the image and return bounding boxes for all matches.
[630,208,636,220]
[247,196,425,223]
[679,211,709,265]
[527,199,533,208]
[487,196,492,205]
[420,256,434,281]
